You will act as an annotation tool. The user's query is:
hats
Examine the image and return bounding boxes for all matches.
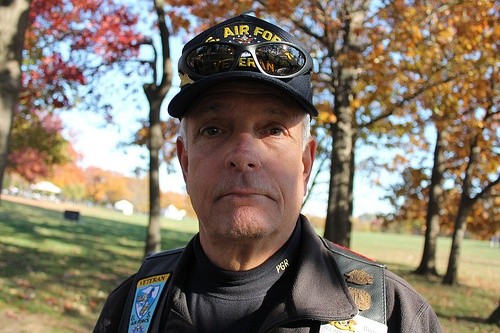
[168,14,319,120]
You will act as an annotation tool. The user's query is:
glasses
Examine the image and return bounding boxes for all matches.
[177,41,315,80]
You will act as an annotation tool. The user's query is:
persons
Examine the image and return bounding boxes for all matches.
[91,12,444,333]
